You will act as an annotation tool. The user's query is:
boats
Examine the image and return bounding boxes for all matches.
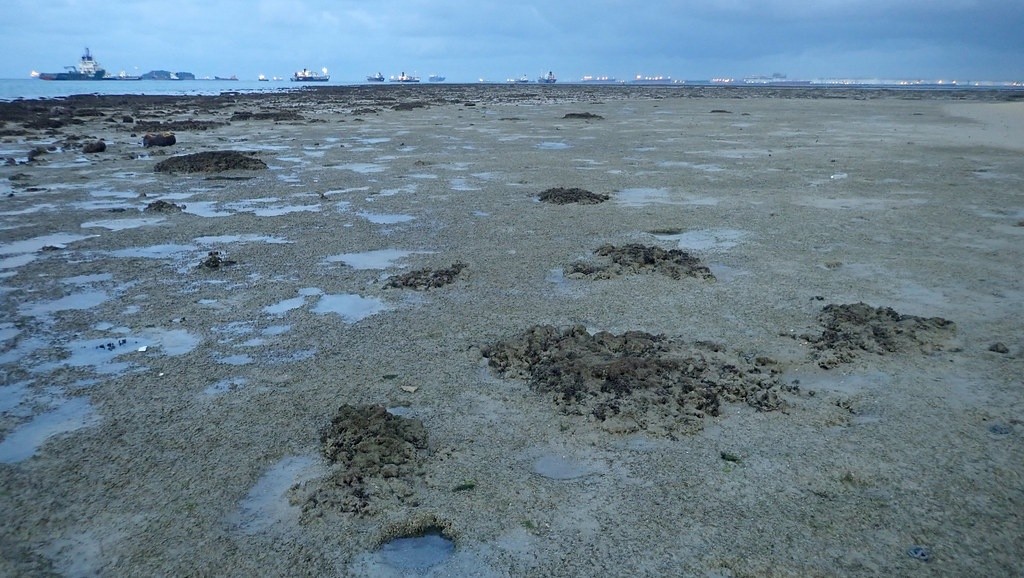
[580,73,625,85]
[102,71,143,81]
[477,78,491,83]
[537,70,557,84]
[215,74,239,81]
[709,76,735,85]
[507,77,514,83]
[367,72,385,82]
[258,72,269,81]
[39,47,106,81]
[624,74,685,86]
[428,74,446,82]
[290,68,331,82]
[743,73,776,84]
[389,72,421,82]
[273,76,283,81]
[516,73,529,83]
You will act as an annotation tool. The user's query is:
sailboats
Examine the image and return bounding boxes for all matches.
[165,72,182,80]
